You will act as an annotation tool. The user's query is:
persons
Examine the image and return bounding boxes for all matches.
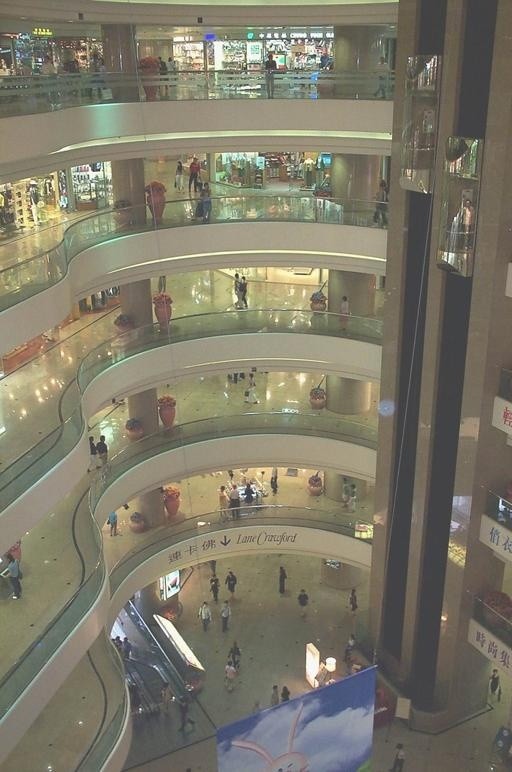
[341,477,350,507]
[458,198,472,252]
[111,634,133,659]
[160,680,173,714]
[390,744,406,772]
[198,600,213,632]
[41,54,176,91]
[281,685,290,703]
[339,295,349,330]
[220,598,231,632]
[251,700,262,714]
[278,566,289,596]
[187,157,202,193]
[227,640,241,671]
[96,436,108,465]
[371,56,393,98]
[107,510,120,536]
[244,372,259,404]
[210,573,222,604]
[229,372,244,384]
[489,668,501,698]
[223,569,238,599]
[158,275,168,293]
[198,181,213,224]
[29,187,41,227]
[3,552,23,600]
[234,273,246,308]
[224,660,237,692]
[240,276,248,308]
[348,588,358,616]
[265,55,277,99]
[220,466,280,523]
[371,178,387,228]
[175,160,188,192]
[296,587,310,617]
[347,483,357,513]
[177,695,197,732]
[343,632,356,661]
[87,436,102,472]
[270,684,279,707]
